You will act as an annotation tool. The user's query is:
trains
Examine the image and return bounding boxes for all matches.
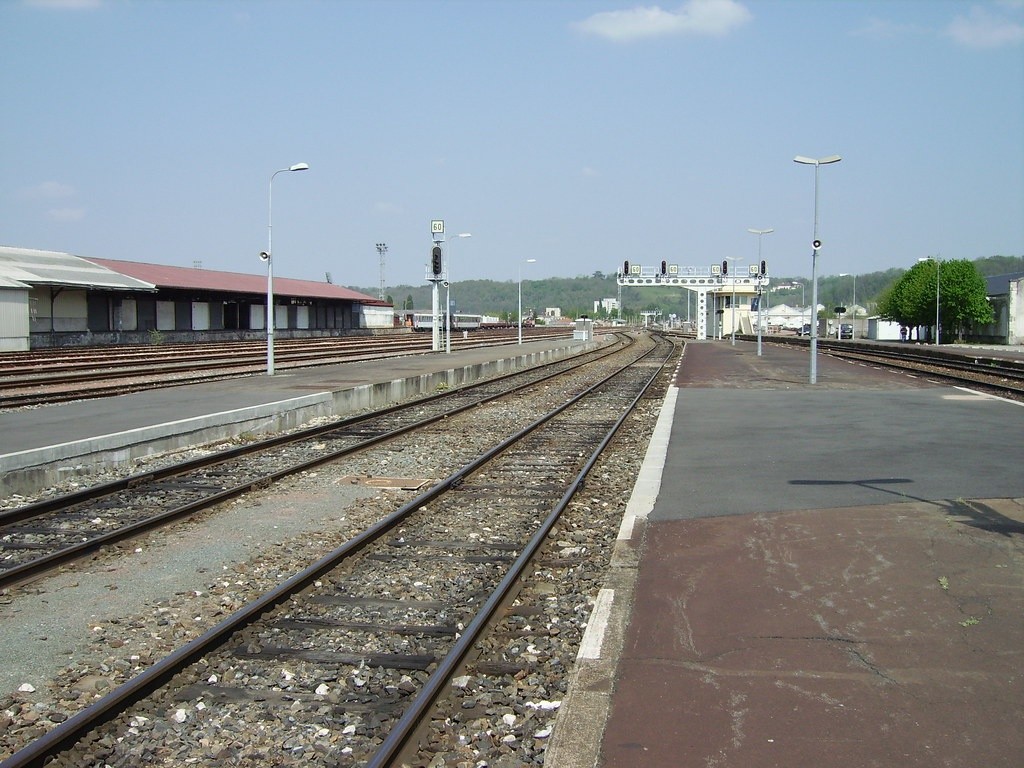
[410,312,534,334]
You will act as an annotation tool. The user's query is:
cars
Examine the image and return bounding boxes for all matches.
[836,325,853,339]
[797,326,811,337]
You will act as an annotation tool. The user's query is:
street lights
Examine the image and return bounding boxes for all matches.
[725,257,744,346]
[792,153,842,383]
[748,228,775,355]
[841,273,855,340]
[259,163,309,374]
[918,257,941,347]
[444,231,472,353]
[517,257,538,345]
[791,281,804,335]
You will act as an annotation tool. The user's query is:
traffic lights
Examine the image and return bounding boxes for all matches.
[760,260,767,275]
[433,246,443,276]
[723,261,728,273]
[662,260,666,275]
[624,261,629,274]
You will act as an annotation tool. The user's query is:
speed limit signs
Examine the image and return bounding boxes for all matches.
[431,220,444,234]
